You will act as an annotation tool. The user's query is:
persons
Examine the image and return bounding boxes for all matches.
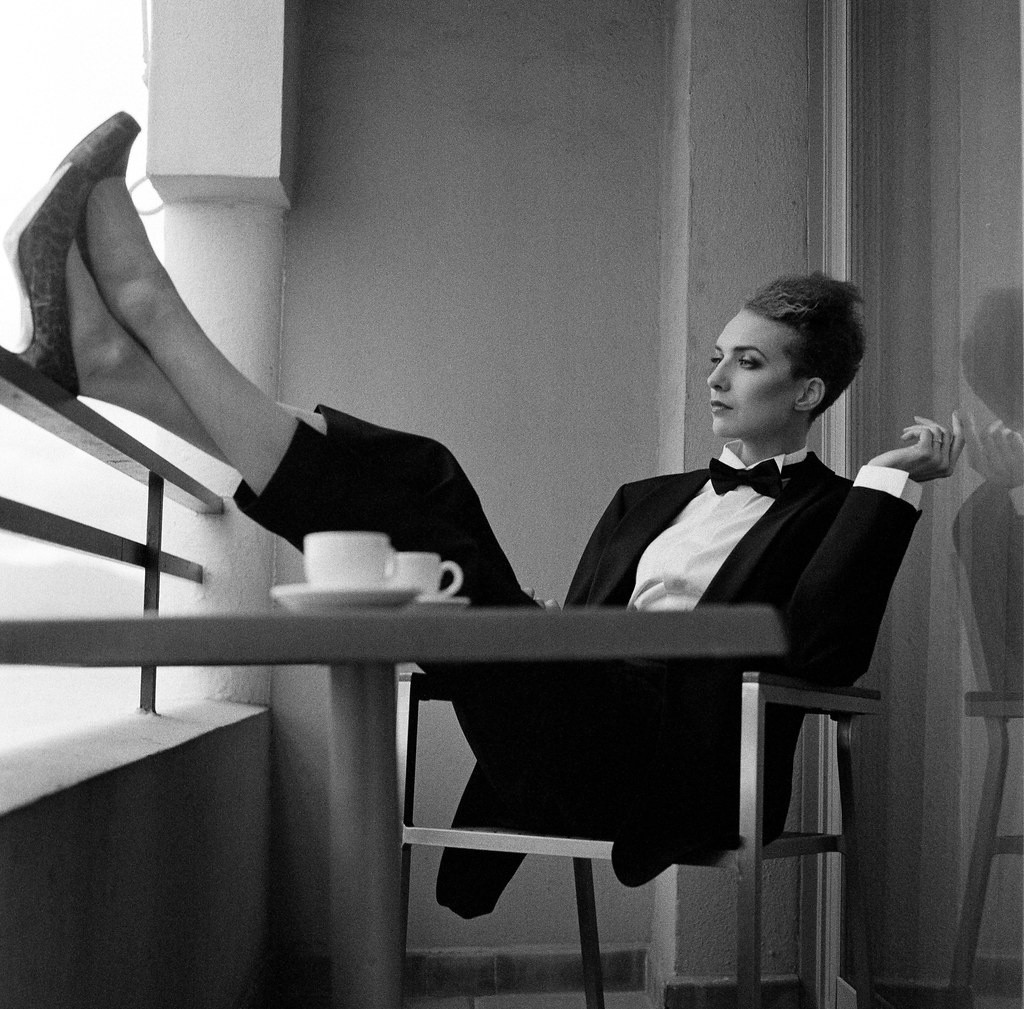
[951,285,1024,699]
[3,112,966,921]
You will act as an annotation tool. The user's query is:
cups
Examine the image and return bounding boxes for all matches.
[387,552,463,596]
[303,532,398,583]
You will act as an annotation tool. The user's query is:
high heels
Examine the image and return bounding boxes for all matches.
[3,161,80,397]
[48,111,142,273]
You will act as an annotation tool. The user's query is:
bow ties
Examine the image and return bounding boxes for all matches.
[709,458,784,499]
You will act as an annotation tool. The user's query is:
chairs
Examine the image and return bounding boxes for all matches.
[396,672,885,1009]
[945,484,1024,1009]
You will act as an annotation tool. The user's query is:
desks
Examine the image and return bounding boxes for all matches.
[0,605,789,1009]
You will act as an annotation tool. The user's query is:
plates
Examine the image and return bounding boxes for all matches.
[414,598,469,610]
[270,582,420,614]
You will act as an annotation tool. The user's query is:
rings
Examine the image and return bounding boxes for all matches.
[932,439,943,443]
